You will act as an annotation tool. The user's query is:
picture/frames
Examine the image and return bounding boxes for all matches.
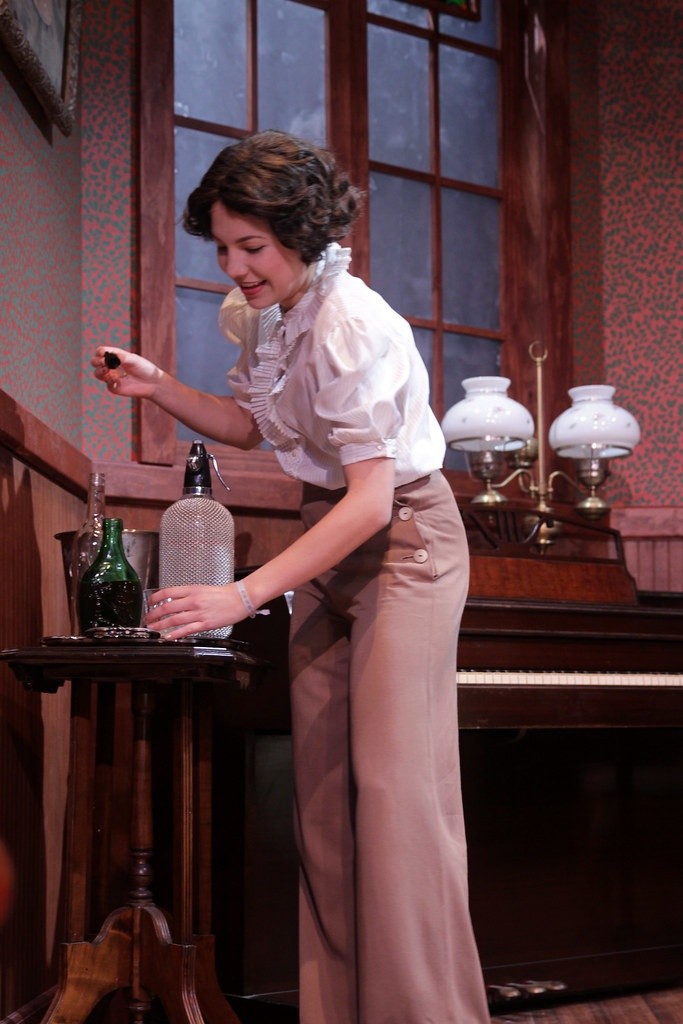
[0,0,85,138]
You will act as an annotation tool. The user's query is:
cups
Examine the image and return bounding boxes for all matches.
[143,589,185,640]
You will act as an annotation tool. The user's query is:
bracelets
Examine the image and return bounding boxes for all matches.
[237,579,256,618]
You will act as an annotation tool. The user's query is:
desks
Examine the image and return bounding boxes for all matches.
[0,645,259,1024]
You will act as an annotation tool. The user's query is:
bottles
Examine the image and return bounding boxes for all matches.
[77,518,142,635]
[66,473,108,634]
[158,439,235,639]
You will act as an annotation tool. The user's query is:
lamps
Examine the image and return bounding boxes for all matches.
[440,340,641,556]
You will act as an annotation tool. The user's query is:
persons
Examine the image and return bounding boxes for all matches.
[91,132,491,1024]
